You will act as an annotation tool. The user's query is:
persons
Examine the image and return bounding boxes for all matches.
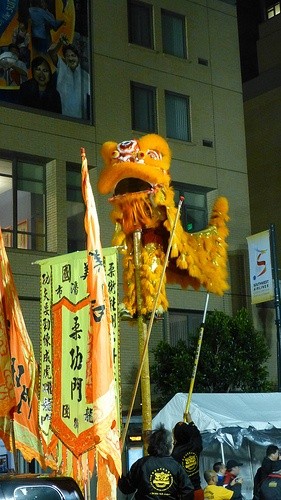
[0,0,90,119]
[171,412,204,500]
[204,444,281,500]
[118,423,194,500]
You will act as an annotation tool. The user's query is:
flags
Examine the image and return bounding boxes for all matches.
[246,230,274,304]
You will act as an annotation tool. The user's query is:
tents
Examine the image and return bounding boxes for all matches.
[147,392,281,500]
